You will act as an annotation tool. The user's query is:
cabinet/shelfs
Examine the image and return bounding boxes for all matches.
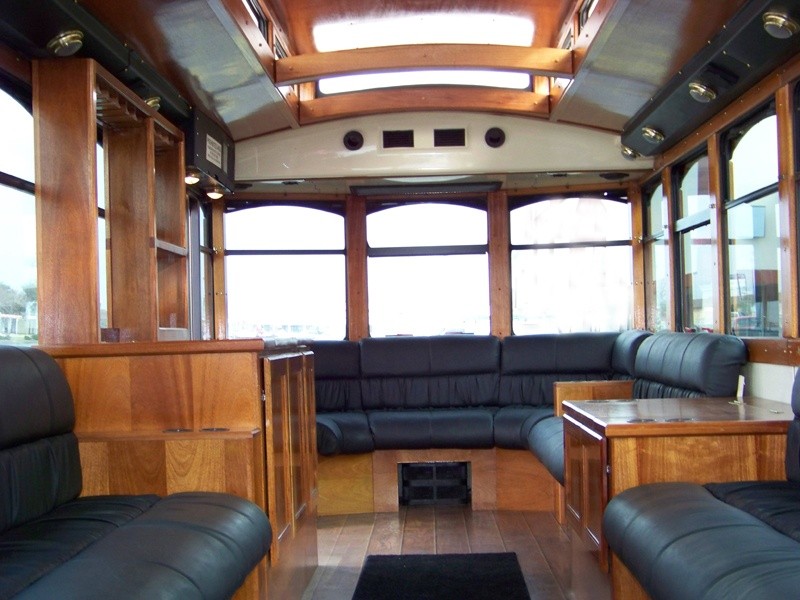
[26,55,324,600]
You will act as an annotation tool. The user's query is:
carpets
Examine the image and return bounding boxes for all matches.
[353,548,532,600]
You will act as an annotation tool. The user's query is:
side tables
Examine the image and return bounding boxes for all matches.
[555,391,794,568]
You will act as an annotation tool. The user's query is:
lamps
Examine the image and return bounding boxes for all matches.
[45,29,85,56]
[180,168,202,185]
[620,144,641,163]
[688,82,717,103]
[641,126,666,148]
[143,93,161,113]
[204,185,224,200]
[762,9,800,38]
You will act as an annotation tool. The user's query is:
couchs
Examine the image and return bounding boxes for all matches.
[0,341,276,600]
[597,366,800,599]
[266,325,751,511]
